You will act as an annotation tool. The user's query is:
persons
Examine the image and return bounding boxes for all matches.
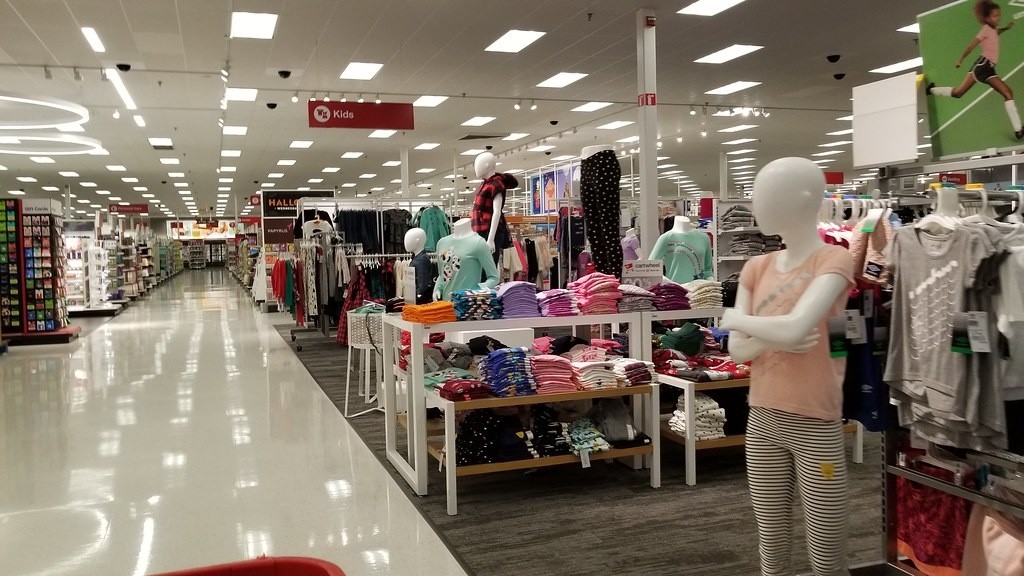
[432,218,500,301]
[387,227,439,313]
[719,157,858,575]
[470,152,514,283]
[649,216,714,285]
[580,144,623,284]
[926,0,1024,139]
[620,228,641,260]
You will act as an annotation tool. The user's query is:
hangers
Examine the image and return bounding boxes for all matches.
[897,437,1023,496]
[819,186,1024,235]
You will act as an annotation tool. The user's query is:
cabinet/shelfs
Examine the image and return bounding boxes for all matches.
[1,197,222,345]
[222,197,786,515]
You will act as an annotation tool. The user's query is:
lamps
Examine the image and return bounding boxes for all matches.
[221,73,230,83]
[497,126,579,160]
[688,104,769,118]
[292,90,381,105]
[42,66,108,81]
[221,63,231,76]
[513,98,522,109]
[529,98,538,110]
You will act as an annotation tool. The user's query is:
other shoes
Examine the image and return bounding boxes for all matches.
[926,82,936,95]
[1015,127,1024,140]
